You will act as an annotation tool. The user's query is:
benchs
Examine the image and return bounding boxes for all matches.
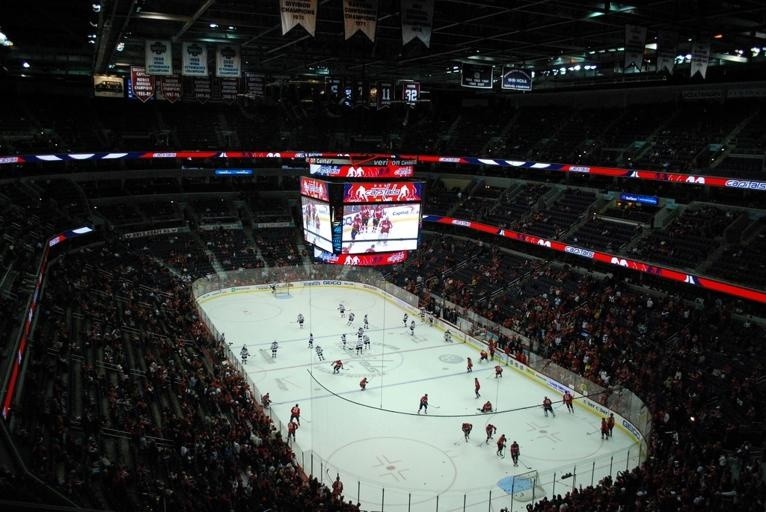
[4,91,765,511]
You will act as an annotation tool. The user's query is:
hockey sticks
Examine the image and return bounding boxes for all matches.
[428,403,440,408]
[587,428,600,435]
[501,442,506,459]
[518,459,531,469]
